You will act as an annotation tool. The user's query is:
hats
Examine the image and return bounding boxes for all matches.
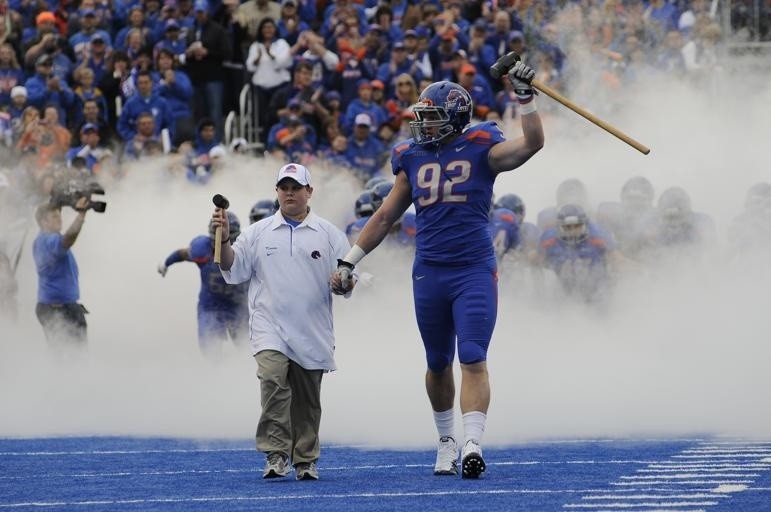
[275,163,313,192]
[84,2,208,43]
[281,5,419,128]
[83,123,99,131]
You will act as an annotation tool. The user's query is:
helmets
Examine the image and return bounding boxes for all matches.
[208,211,241,243]
[491,177,692,245]
[248,201,273,224]
[354,177,395,218]
[408,81,473,148]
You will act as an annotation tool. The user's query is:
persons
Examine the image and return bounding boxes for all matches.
[32,198,87,354]
[333,61,544,478]
[0,1,770,289]
[157,213,248,362]
[209,164,355,480]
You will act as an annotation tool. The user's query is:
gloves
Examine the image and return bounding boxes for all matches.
[332,261,357,299]
[509,61,536,100]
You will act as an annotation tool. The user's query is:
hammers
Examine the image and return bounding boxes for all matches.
[491,51,650,155]
[213,194,229,264]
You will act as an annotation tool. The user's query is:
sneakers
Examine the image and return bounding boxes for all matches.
[263,455,291,478]
[462,441,486,477]
[434,437,459,474]
[295,462,319,481]
